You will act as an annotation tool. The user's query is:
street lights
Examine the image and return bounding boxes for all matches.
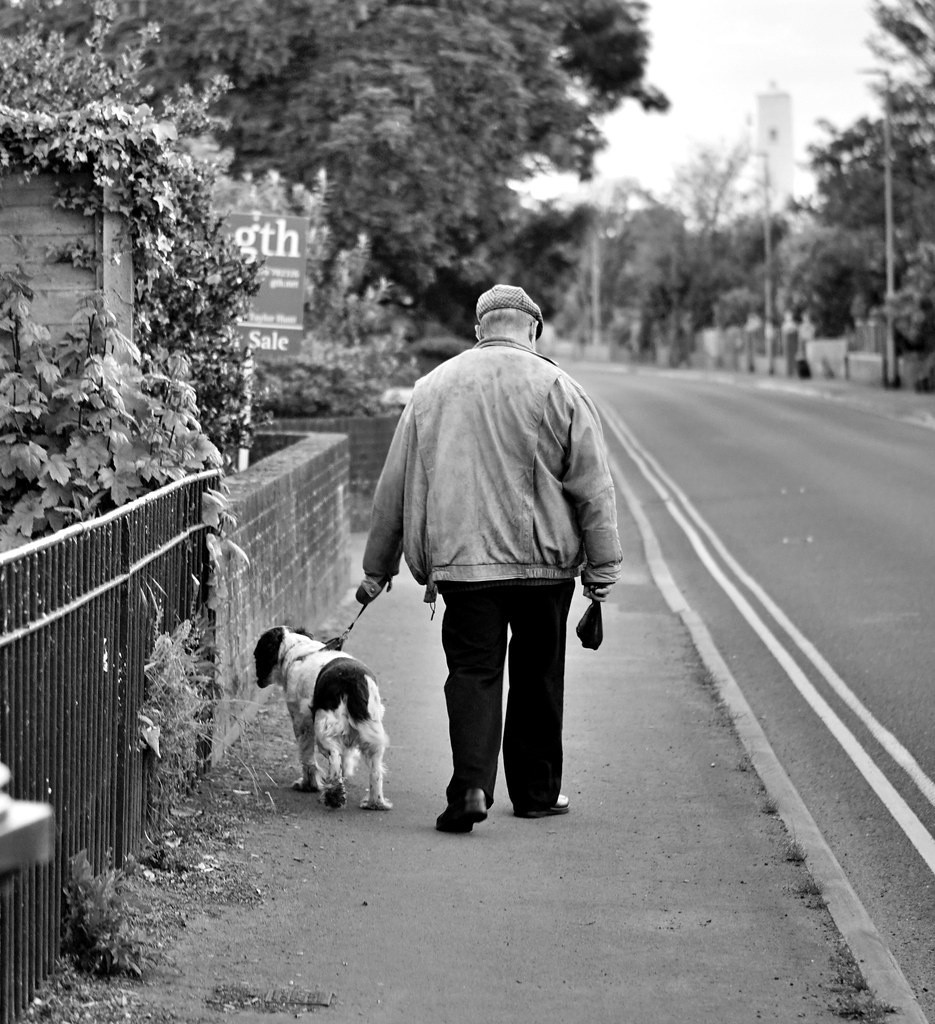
[860,64,898,387]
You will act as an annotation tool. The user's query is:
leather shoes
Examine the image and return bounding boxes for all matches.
[514,793,571,818]
[436,787,488,834]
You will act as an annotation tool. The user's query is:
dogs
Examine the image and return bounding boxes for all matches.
[252,626,394,812]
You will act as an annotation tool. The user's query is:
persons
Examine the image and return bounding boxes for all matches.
[356,285,624,836]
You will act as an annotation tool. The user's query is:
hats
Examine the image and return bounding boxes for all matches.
[477,283,545,340]
